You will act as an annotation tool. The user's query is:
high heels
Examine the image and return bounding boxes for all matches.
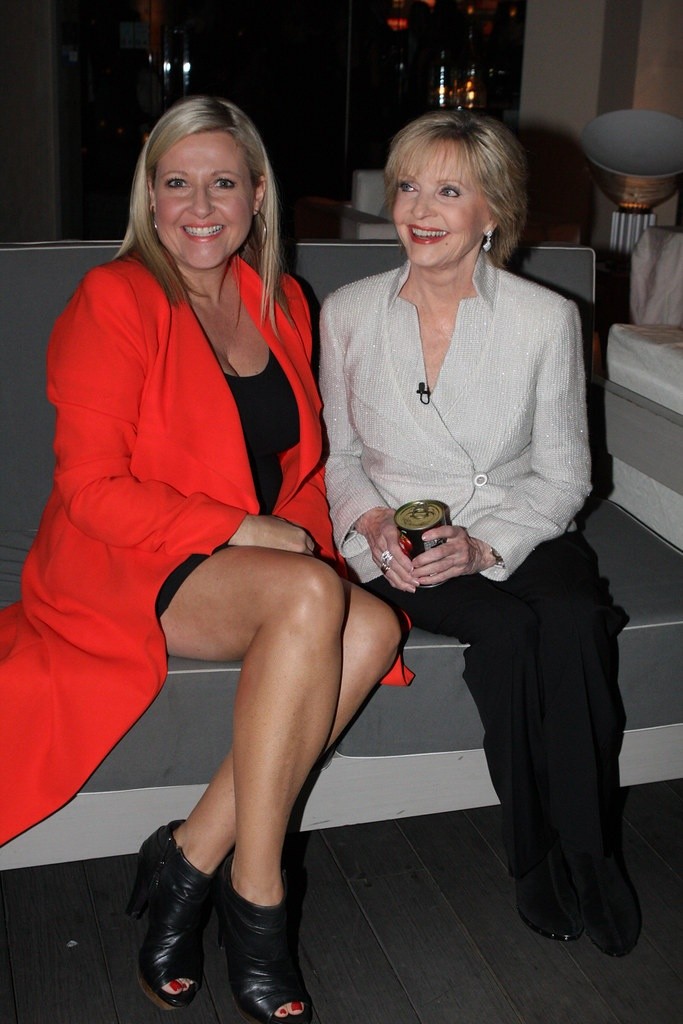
[216,852,315,1024]
[124,819,218,1011]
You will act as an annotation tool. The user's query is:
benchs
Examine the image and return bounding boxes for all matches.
[0,237,683,872]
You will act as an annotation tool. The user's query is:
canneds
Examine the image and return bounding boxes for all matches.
[394,499,451,588]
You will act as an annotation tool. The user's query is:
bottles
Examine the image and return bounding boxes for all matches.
[433,45,486,110]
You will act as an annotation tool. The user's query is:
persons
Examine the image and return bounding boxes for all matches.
[0,95,415,1024]
[320,109,645,959]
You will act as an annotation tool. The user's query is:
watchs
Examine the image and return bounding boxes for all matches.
[491,547,504,565]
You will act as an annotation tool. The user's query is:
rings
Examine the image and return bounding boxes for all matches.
[381,550,393,575]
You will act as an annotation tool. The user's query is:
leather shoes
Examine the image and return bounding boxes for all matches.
[514,843,584,942]
[559,837,641,957]
